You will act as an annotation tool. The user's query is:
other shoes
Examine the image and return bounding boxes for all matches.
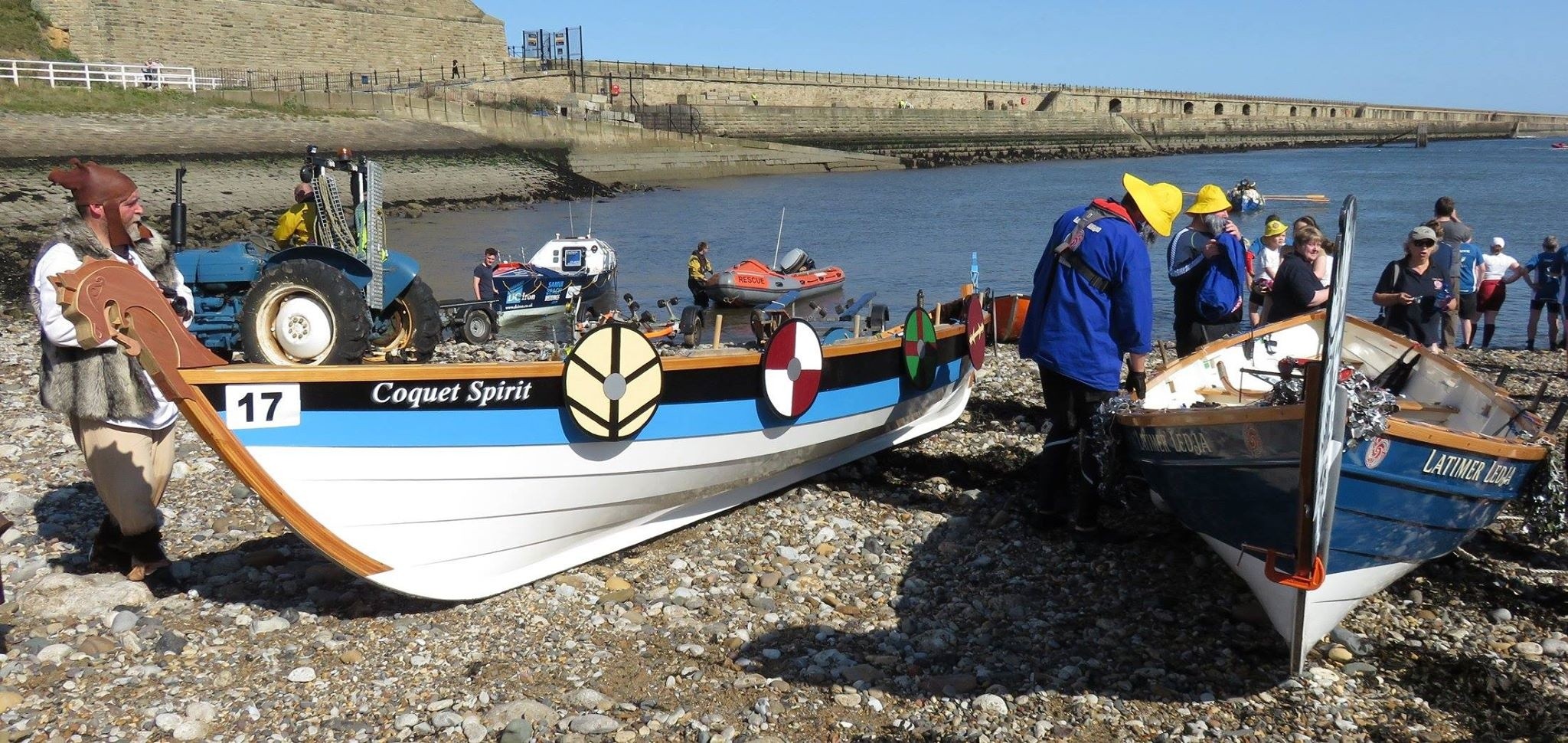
[88,523,123,564]
[1458,343,1469,349]
[135,547,181,598]
[1525,339,1534,351]
[1038,485,1097,531]
[1549,341,1557,350]
[1482,346,1488,350]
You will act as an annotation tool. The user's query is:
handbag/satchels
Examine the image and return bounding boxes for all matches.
[1373,312,1388,329]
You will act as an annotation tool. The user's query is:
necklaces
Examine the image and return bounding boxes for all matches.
[104,244,136,266]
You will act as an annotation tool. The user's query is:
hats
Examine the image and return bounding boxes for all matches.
[1490,237,1505,248]
[48,159,138,248]
[1409,226,1436,242]
[1264,220,1289,237]
[1123,173,1183,238]
[1184,184,1232,217]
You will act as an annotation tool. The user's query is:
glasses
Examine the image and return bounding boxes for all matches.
[1414,239,1434,246]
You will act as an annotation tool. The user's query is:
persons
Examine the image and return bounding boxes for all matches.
[1457,227,1486,350]
[1292,214,1335,287]
[1549,243,1568,351]
[272,182,319,250]
[1165,183,1249,360]
[1372,226,1458,356]
[1017,171,1184,533]
[451,59,461,79]
[1521,234,1561,353]
[28,157,200,582]
[687,241,721,310]
[141,57,167,90]
[1251,220,1289,296]
[1259,224,1329,327]
[540,57,553,72]
[1417,218,1454,346]
[1245,214,1281,330]
[1231,176,1257,200]
[1431,195,1471,354]
[472,247,499,341]
[1468,236,1524,350]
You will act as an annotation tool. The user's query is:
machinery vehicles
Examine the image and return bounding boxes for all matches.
[160,145,443,367]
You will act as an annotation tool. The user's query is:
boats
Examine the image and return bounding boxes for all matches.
[46,257,991,600]
[487,234,618,326]
[704,249,845,305]
[1552,141,1568,148]
[1107,194,1547,682]
[984,294,1032,343]
